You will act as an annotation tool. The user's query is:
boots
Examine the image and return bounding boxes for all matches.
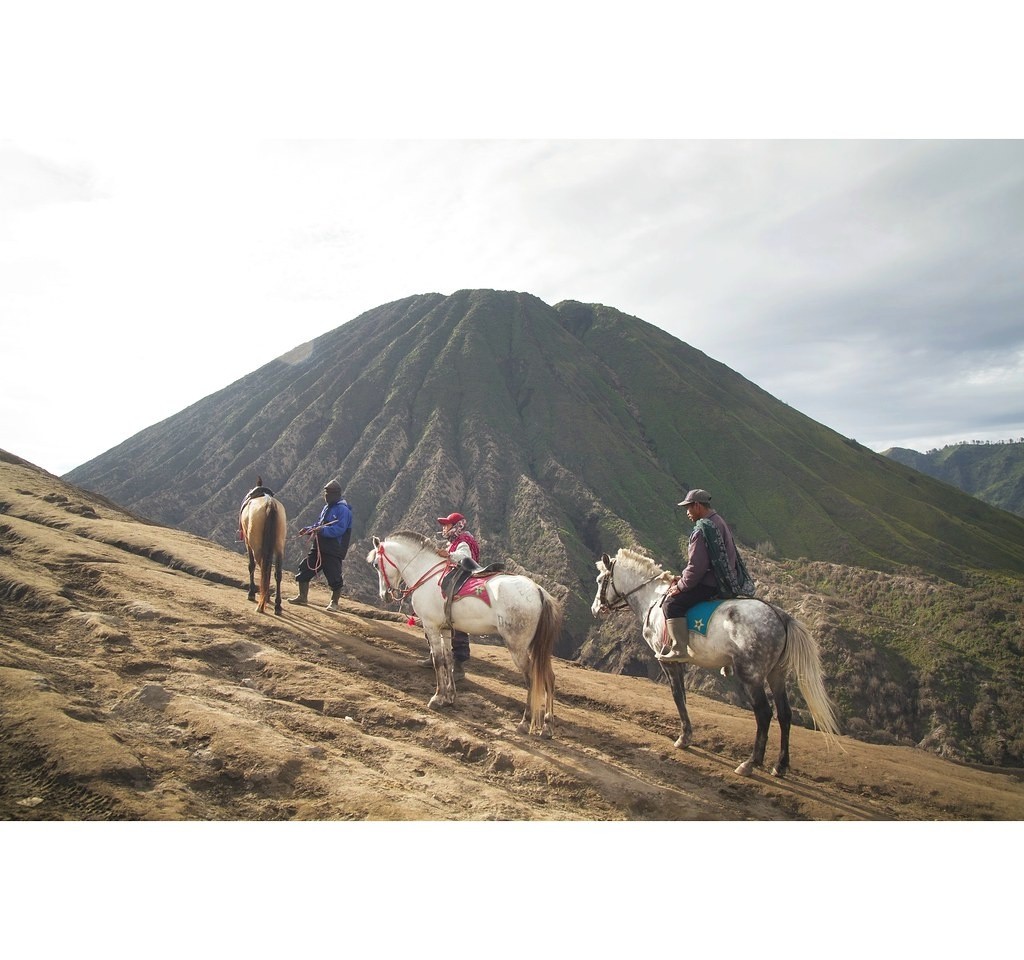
[451,661,465,682]
[287,581,308,606]
[658,618,690,663]
[417,652,433,667]
[327,587,341,612]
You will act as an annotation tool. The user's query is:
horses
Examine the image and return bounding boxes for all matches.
[367,530,562,740]
[238,492,288,615]
[591,548,851,777]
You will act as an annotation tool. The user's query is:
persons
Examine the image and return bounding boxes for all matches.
[657,488,755,663]
[417,512,479,681]
[286,480,353,612]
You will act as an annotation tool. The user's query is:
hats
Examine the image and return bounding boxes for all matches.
[438,513,464,526]
[677,489,712,507]
[323,480,341,494]
